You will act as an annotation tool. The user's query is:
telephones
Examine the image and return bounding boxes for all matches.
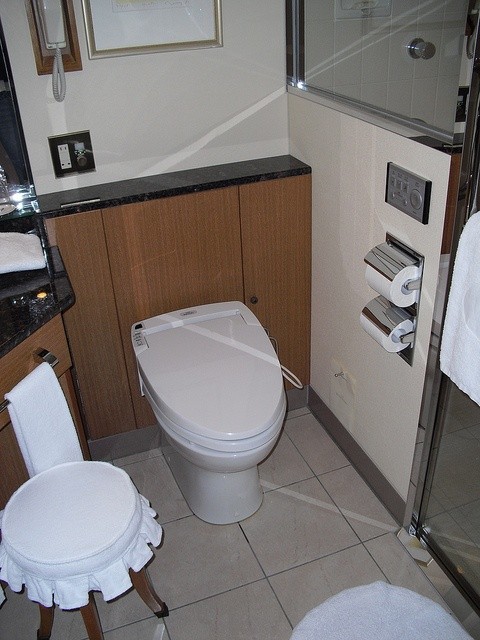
[30,1,69,102]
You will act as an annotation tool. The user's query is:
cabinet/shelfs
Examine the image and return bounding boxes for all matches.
[241,171,311,392]
[41,184,242,442]
[1,313,87,514]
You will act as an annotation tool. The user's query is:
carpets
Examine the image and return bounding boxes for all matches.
[286,579,473,640]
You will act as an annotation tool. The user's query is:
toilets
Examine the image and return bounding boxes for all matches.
[129,298,291,525]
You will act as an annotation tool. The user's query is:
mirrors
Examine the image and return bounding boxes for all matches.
[284,0,480,155]
[81,0,224,62]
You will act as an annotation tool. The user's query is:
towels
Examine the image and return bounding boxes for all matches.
[4,360,84,479]
[0,230,50,275]
[436,209,480,409]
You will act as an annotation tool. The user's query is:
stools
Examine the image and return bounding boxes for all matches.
[0,460,168,640]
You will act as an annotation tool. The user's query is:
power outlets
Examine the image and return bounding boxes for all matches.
[48,127,97,181]
